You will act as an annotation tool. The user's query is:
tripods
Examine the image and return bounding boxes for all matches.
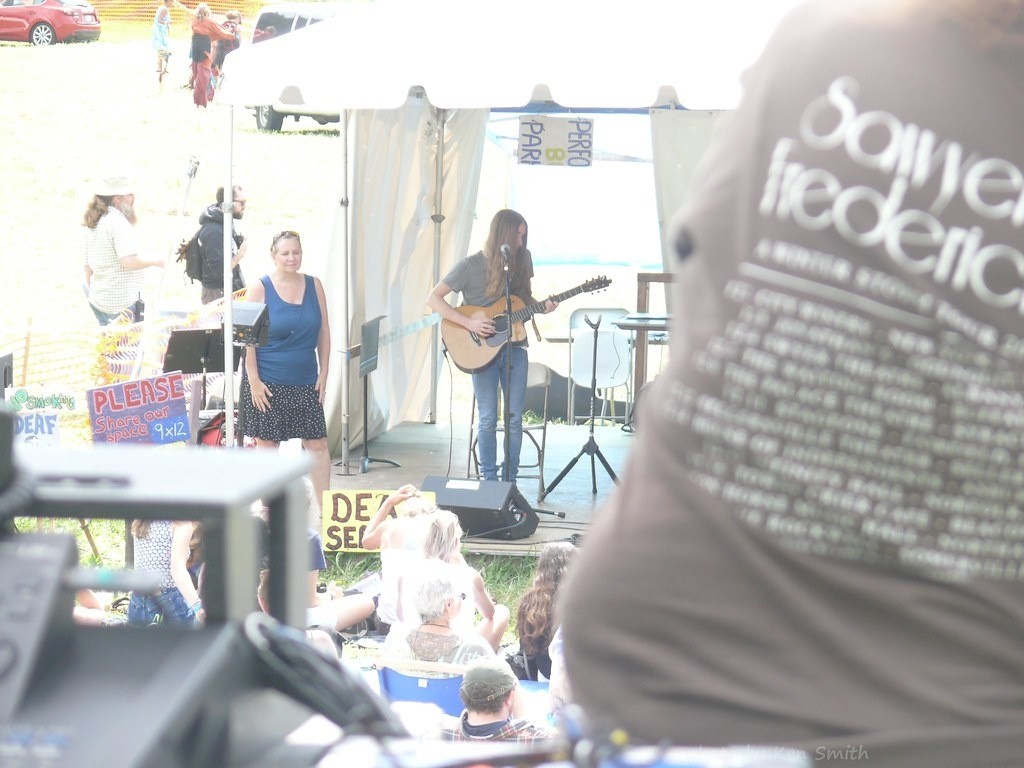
[330,315,402,474]
[538,314,620,504]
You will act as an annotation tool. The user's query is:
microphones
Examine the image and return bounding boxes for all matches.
[500,243,511,260]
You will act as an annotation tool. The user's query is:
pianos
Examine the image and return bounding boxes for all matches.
[613,309,670,432]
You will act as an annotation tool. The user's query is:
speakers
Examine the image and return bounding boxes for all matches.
[421,475,541,542]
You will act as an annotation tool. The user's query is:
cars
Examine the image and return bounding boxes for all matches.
[214,0,763,131]
[1,433,320,768]
[0,0,102,46]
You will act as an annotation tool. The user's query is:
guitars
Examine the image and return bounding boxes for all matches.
[440,274,612,373]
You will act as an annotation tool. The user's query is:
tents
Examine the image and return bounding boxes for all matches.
[221,0,786,475]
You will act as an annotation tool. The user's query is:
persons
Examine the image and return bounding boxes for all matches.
[84,178,169,326]
[77,474,574,744]
[197,185,246,304]
[152,0,277,108]
[426,210,559,486]
[561,0,1024,768]
[241,231,332,519]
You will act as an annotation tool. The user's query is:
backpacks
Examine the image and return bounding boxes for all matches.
[176,220,216,285]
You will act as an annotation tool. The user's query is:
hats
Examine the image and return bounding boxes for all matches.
[94,168,134,197]
[462,654,515,700]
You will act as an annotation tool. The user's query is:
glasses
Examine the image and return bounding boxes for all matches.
[460,593,466,600]
[271,230,300,250]
[232,200,246,207]
